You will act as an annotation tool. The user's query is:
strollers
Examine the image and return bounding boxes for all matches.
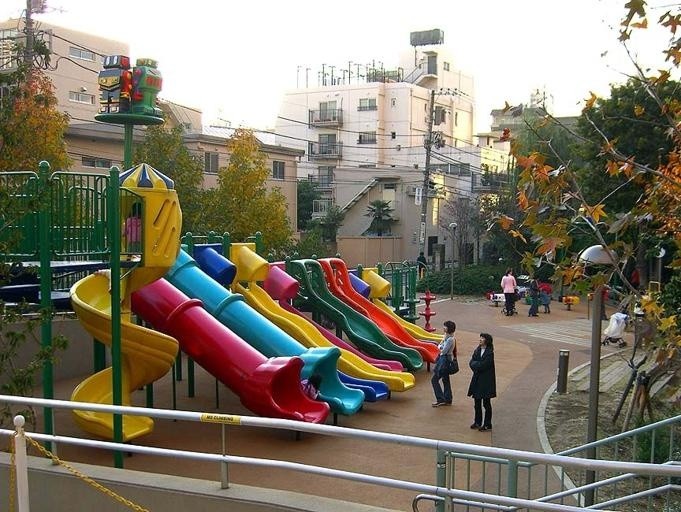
[500,289,520,316]
[601,313,628,348]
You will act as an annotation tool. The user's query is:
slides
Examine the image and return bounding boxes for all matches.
[70,268,180,444]
[234,282,416,392]
[164,246,365,416]
[316,258,441,364]
[350,269,446,351]
[278,299,403,372]
[131,275,330,426]
[289,258,424,372]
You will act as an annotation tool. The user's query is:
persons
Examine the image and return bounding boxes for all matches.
[500,266,519,317]
[125,202,143,257]
[592,271,610,321]
[631,264,641,286]
[466,331,498,432]
[542,289,551,315]
[301,373,324,400]
[527,273,538,317]
[417,250,428,280]
[632,289,651,319]
[430,319,460,408]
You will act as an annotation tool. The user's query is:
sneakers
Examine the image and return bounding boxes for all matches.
[479,424,492,431]
[471,422,480,428]
[432,400,445,407]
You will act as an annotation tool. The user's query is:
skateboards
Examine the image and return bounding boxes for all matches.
[240,297,391,403]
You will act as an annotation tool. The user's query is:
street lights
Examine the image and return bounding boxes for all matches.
[449,223,457,298]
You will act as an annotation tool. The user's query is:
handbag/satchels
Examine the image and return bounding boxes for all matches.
[445,358,459,374]
